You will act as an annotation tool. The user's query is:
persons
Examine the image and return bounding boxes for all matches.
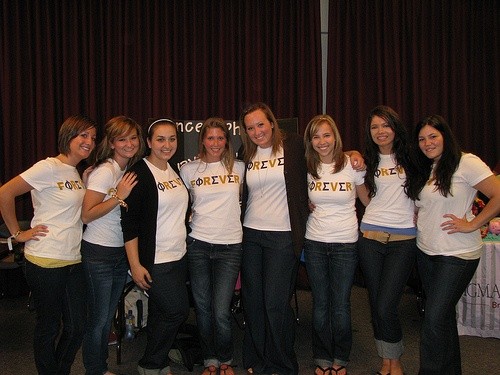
[240,102,367,375]
[82,116,146,375]
[0,114,98,375]
[412,114,500,375]
[303,114,370,375]
[307,106,414,375]
[120,116,192,375]
[180,118,245,375]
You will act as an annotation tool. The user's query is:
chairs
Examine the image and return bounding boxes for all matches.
[113,249,310,372]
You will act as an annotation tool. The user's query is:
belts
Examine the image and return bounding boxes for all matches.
[361,229,415,244]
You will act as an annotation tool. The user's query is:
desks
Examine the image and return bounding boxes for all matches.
[456,234,499,339]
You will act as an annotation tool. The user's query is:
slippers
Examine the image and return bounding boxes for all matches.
[315,363,331,375]
[329,365,347,375]
[202,366,217,375]
[219,364,234,375]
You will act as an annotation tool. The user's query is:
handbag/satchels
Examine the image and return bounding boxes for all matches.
[121,290,148,330]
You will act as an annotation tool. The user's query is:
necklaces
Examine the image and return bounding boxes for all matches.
[258,150,271,198]
